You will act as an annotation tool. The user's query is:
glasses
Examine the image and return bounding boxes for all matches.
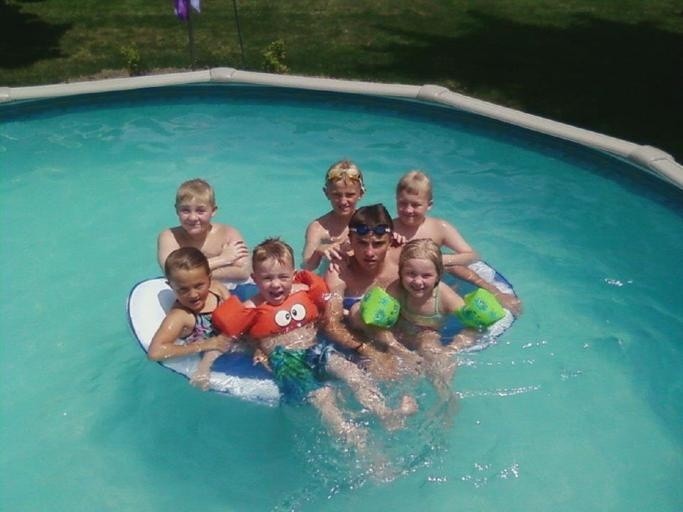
[350,224,391,234]
